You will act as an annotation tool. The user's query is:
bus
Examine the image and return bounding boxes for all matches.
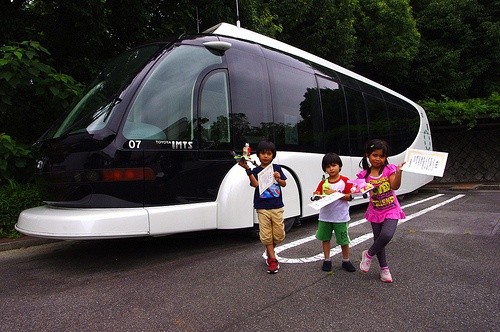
[15,22,435,242]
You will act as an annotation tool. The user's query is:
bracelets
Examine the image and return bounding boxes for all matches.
[246,168,253,176]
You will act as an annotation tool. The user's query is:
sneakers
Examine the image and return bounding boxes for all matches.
[360,250,372,273]
[266,258,270,266]
[267,258,280,273]
[379,268,392,283]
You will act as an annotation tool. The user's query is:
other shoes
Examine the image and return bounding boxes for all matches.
[323,261,331,271]
[342,261,355,272]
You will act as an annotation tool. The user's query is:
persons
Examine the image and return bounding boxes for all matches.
[312,152,356,272]
[238,141,287,274]
[356,139,406,283]
